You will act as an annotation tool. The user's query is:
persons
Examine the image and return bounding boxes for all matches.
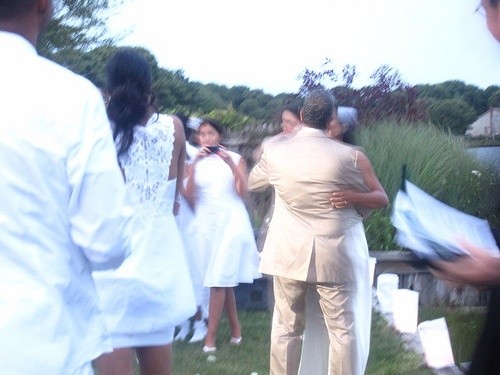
[247,89,389,375]
[93,49,198,375]
[0,0,135,375]
[173,112,263,353]
[431,0,500,375]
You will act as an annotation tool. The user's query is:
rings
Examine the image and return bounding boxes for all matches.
[344,201,347,205]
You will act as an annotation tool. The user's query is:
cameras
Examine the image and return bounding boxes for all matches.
[207,146,219,153]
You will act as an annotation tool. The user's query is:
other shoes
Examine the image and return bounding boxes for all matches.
[230,336,243,345]
[187,320,209,343]
[173,320,192,341]
[203,345,217,353]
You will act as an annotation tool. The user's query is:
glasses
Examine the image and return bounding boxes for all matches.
[474,0,489,16]
